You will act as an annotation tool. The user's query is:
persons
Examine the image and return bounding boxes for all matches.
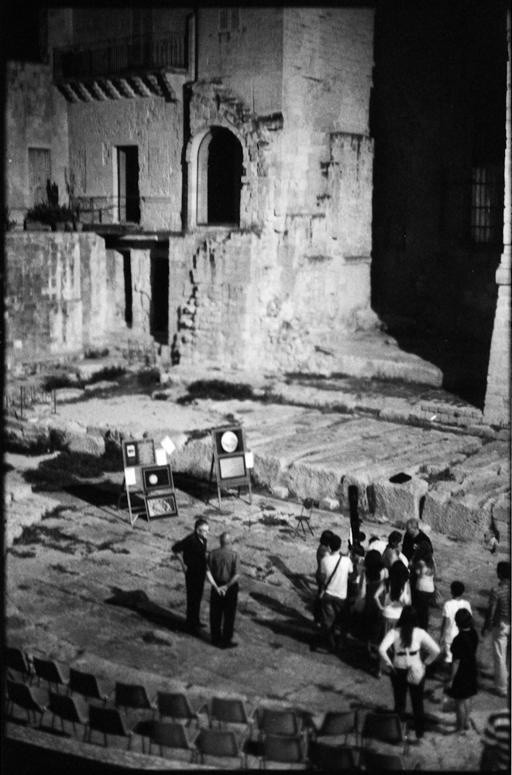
[377,581,480,743]
[316,519,436,650]
[172,519,209,633]
[482,560,512,697]
[206,533,241,648]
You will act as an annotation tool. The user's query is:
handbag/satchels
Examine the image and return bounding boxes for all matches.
[407,661,427,686]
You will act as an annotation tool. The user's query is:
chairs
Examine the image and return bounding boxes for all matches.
[4,646,403,772]
[293,497,315,539]
